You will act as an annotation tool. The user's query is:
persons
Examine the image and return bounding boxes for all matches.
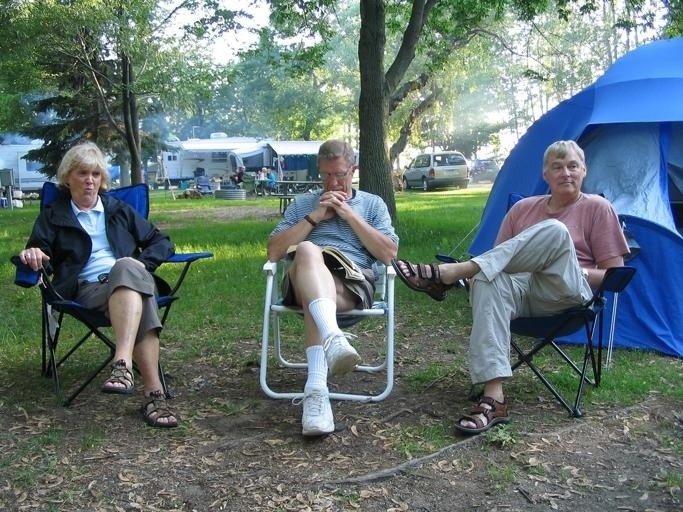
[179,164,274,201]
[18,142,179,427]
[266,140,399,435]
[392,140,630,435]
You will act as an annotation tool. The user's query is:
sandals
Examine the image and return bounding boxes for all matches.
[453,397,510,434]
[100,361,136,393]
[389,256,453,301]
[139,389,178,428]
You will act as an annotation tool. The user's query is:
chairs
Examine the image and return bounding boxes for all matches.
[14,185,214,408]
[259,229,398,403]
[435,194,637,418]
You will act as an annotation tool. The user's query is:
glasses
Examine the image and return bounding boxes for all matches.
[317,166,352,181]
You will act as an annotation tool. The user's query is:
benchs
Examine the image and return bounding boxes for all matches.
[255,180,323,215]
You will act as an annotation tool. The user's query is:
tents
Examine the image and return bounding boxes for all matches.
[464,32,681,360]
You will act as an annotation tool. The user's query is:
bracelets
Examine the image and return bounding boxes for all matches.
[304,216,317,228]
[582,266,588,282]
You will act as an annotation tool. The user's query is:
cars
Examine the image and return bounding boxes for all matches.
[470,160,501,182]
[402,151,470,190]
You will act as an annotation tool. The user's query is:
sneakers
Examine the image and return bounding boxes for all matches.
[300,387,335,437]
[321,333,360,376]
